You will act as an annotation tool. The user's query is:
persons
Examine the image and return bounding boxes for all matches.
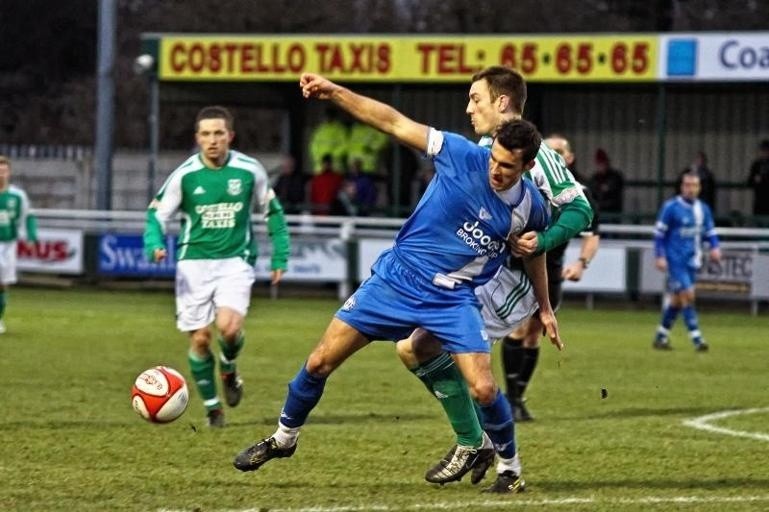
[746,139,767,226]
[397,65,594,482]
[143,105,292,427]
[271,137,437,227]
[589,148,624,238]
[674,152,716,213]
[653,172,720,350]
[234,72,564,495]
[502,135,601,421]
[0,156,38,333]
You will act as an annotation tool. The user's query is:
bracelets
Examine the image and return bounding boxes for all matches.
[578,258,590,269]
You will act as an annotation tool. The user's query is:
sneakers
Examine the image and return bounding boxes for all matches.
[469,447,497,486]
[654,330,671,351]
[692,335,711,352]
[424,430,496,486]
[232,435,298,473]
[205,408,226,428]
[480,468,528,495]
[222,370,245,408]
[509,396,535,423]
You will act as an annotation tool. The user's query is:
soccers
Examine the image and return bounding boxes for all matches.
[131,366,189,423]
